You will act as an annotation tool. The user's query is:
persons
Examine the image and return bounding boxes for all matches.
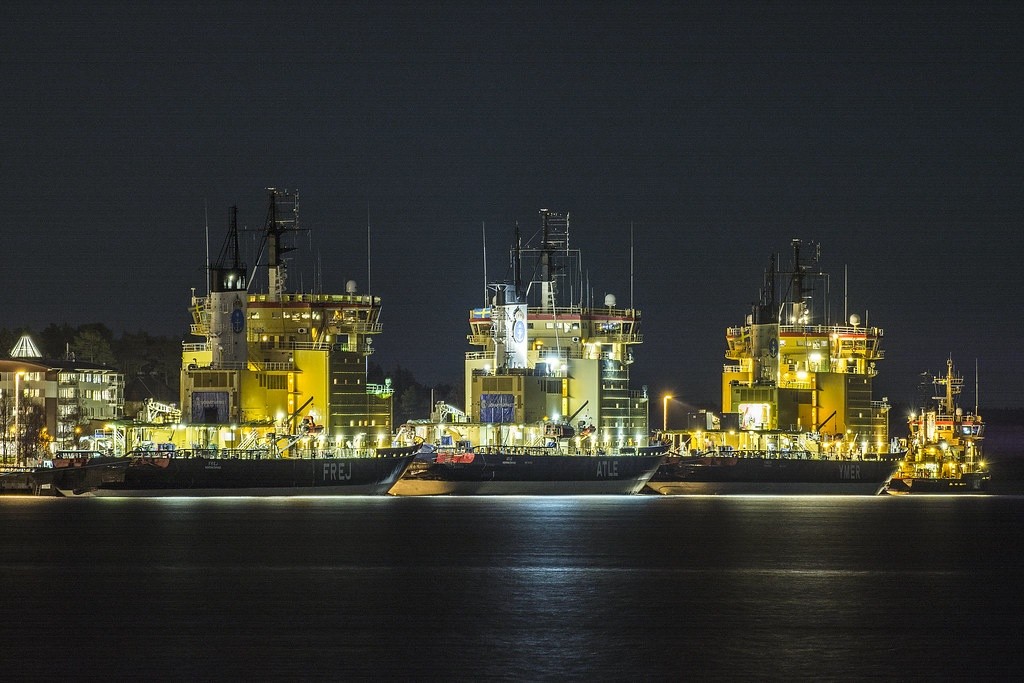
[464,444,608,456]
[652,429,903,460]
[57,418,418,459]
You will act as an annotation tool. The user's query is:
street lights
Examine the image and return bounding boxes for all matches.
[663,392,673,432]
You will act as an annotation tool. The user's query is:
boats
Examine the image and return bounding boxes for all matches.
[646,238,909,497]
[881,352,992,498]
[52,187,429,499]
[386,208,674,498]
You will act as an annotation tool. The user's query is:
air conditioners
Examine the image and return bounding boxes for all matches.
[298,327,307,333]
[572,337,579,342]
